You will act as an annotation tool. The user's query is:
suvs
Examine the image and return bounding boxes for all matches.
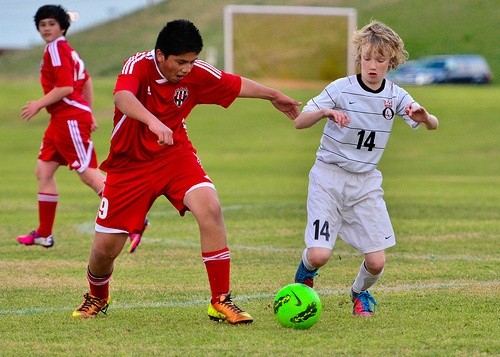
[402,55,494,87]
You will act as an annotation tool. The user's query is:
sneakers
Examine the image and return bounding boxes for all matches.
[206,293,254,325]
[71,291,110,319]
[16,230,53,247]
[295,261,317,289]
[350,287,376,316]
[127,218,148,253]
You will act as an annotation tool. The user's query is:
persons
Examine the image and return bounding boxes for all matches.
[70,19,301,323]
[296,20,439,320]
[17,4,149,253]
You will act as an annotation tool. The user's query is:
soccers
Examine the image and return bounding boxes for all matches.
[274,283,322,329]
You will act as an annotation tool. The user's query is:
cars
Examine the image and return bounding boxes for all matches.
[385,61,417,84]
